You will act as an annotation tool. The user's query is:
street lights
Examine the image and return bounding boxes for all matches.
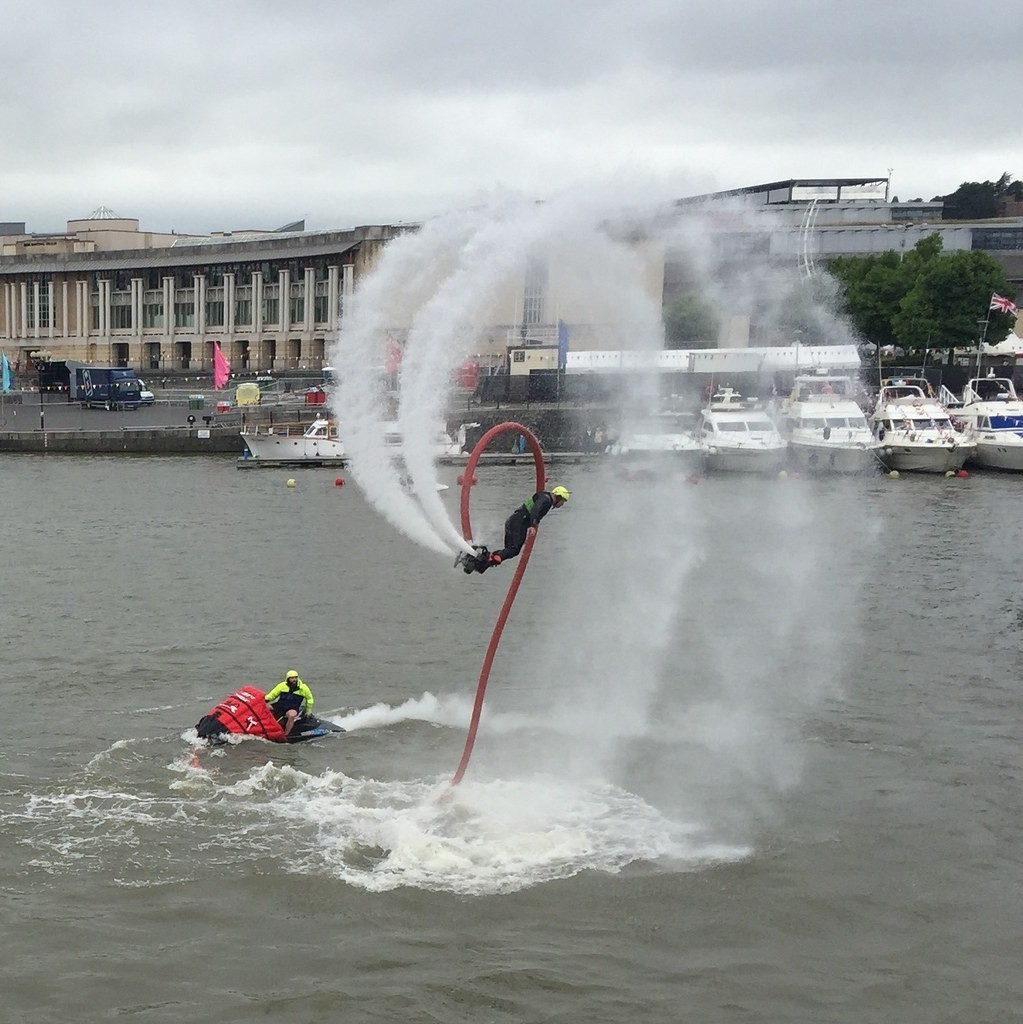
[31,349,52,430]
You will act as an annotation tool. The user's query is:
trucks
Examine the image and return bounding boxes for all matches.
[69,367,143,412]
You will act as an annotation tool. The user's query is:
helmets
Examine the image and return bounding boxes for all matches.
[552,486,569,501]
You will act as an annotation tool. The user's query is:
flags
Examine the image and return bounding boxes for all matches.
[3,353,10,393]
[990,292,1017,316]
[214,340,231,391]
[559,320,570,366]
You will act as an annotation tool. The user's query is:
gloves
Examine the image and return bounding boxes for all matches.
[304,712,311,718]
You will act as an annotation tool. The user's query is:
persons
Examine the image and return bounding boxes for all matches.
[265,670,313,736]
[584,425,617,455]
[454,486,570,575]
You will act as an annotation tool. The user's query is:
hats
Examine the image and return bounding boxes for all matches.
[286,670,298,681]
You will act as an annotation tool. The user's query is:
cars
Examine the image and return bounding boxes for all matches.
[137,378,155,408]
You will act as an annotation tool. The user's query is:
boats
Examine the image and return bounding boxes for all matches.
[693,396,791,474]
[601,427,706,486]
[870,387,978,474]
[943,376,1023,480]
[432,444,599,471]
[778,377,885,475]
[240,414,468,479]
